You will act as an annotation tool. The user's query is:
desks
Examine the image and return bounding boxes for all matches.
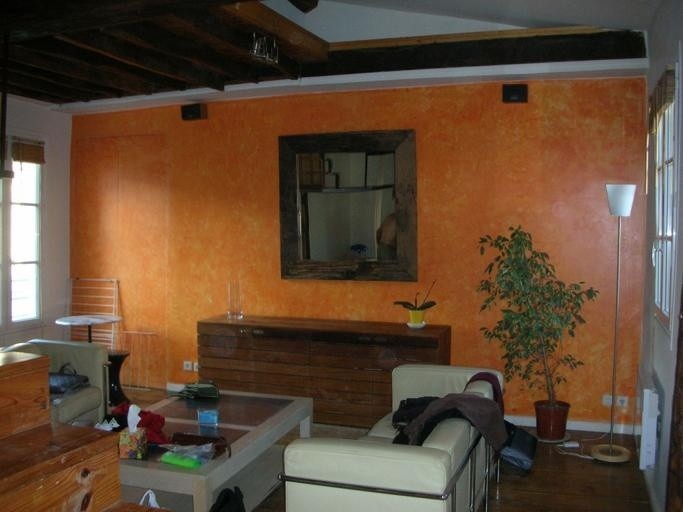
[56,314,125,342]
[195,313,451,431]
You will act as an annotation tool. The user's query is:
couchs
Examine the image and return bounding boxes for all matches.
[281,365,499,512]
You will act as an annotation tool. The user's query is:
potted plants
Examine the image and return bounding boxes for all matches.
[476,224,599,442]
[392,298,437,330]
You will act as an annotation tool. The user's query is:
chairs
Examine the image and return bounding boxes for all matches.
[4,338,109,424]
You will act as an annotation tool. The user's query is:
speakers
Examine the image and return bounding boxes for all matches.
[180,103,208,121]
[502,84,528,103]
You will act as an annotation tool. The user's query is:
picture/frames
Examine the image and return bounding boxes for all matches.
[276,129,418,286]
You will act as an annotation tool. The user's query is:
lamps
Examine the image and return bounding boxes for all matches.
[592,181,636,465]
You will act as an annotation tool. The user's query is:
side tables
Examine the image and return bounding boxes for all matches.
[105,350,133,406]
